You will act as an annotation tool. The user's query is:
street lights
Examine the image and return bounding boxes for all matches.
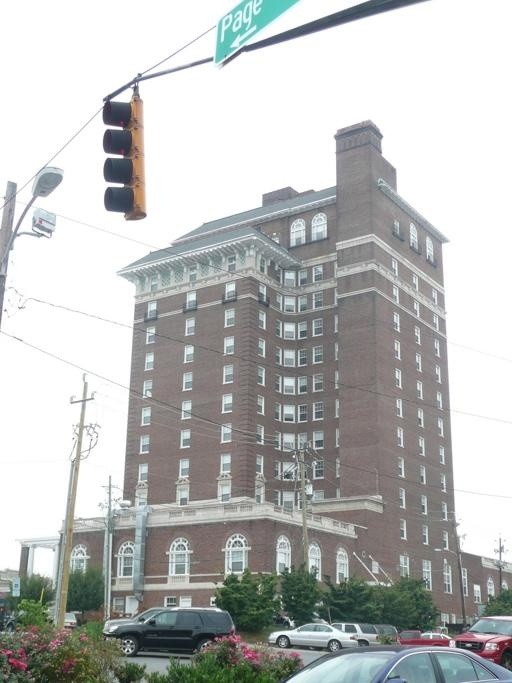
[0,165,64,325]
[104,501,132,619]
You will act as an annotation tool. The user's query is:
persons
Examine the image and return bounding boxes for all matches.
[466,624,472,630]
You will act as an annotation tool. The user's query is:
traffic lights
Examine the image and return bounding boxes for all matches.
[103,100,133,212]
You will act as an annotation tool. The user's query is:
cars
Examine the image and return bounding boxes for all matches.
[102,606,171,636]
[279,643,512,681]
[268,615,452,650]
[1,613,17,631]
[52,612,77,628]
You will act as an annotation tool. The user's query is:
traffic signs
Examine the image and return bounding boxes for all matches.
[213,0,299,66]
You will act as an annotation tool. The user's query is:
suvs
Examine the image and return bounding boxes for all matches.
[449,615,512,670]
[108,608,235,656]
